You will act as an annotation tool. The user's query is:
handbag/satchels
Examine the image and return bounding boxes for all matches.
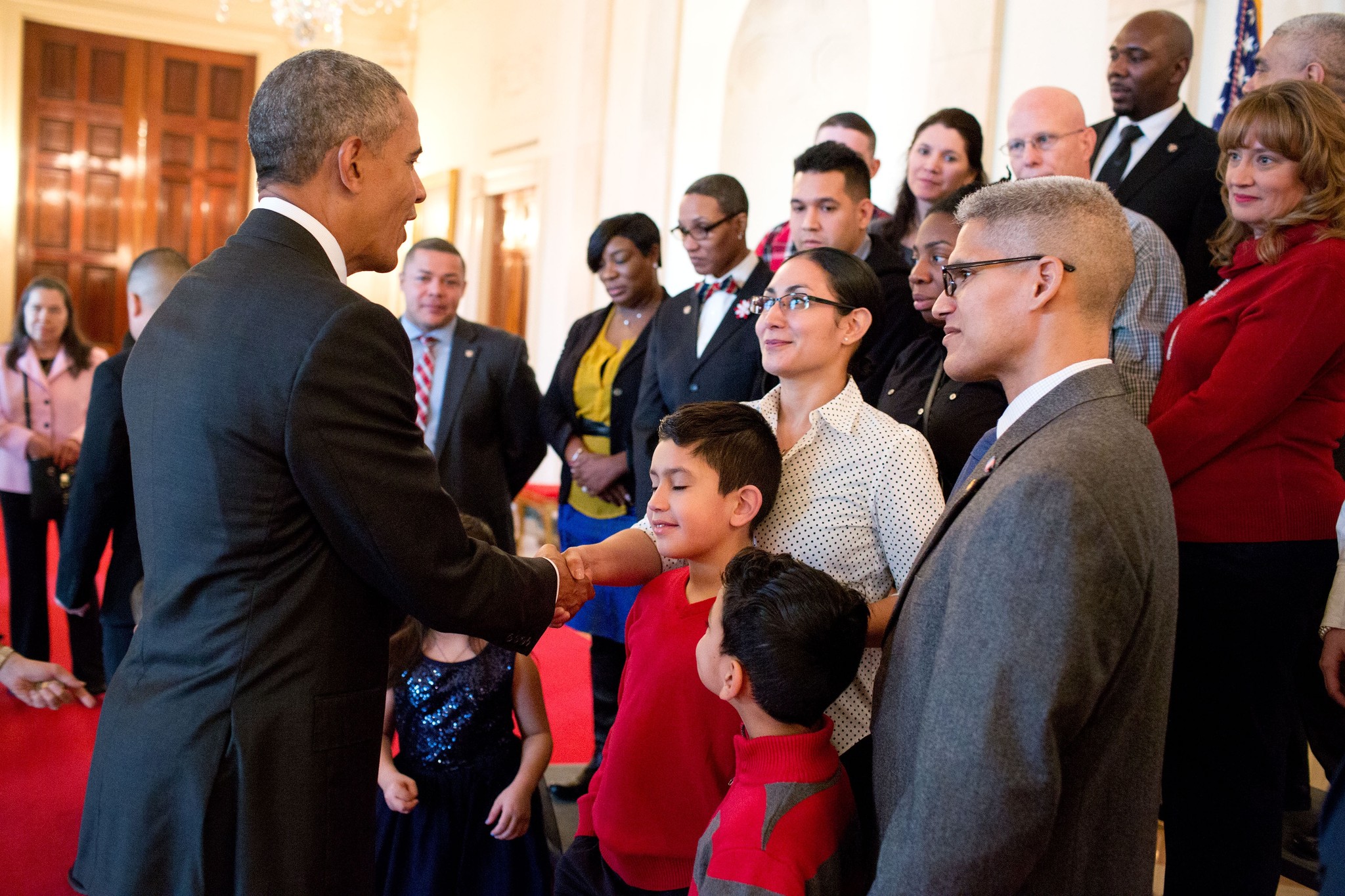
[29,456,78,514]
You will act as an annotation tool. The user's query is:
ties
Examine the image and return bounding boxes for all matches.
[410,335,438,439]
[1095,126,1144,194]
[948,426,997,505]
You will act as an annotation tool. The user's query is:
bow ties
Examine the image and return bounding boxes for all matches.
[695,276,740,304]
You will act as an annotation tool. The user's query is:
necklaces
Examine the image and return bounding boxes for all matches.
[624,314,643,325]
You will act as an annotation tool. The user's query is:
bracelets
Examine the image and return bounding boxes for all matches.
[0,646,14,667]
[572,447,584,461]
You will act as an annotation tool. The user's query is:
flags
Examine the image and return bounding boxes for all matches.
[1210,0,1261,134]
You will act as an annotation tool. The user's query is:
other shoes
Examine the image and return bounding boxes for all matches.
[551,781,589,804]
[1278,834,1320,889]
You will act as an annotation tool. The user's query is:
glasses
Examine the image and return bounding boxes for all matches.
[998,128,1084,158]
[943,254,1078,298]
[672,209,745,244]
[749,292,858,316]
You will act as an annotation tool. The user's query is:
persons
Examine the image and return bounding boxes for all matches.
[69,52,594,896]
[863,179,1179,896]
[52,246,190,691]
[0,277,108,697]
[688,546,873,896]
[0,634,97,710]
[375,9,1345,896]
